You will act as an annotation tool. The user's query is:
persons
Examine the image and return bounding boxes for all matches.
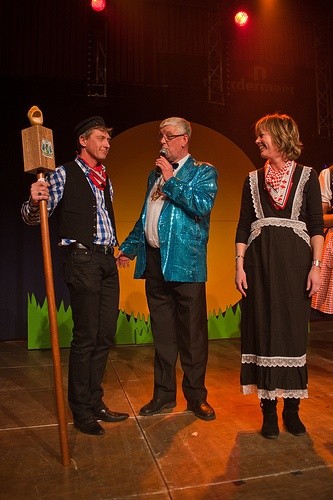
[234,114,326,438]
[115,116,215,421]
[311,164,333,314]
[21,117,129,435]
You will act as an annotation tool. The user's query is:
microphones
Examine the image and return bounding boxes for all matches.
[155,148,167,177]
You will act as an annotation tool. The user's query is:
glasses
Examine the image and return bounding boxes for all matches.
[158,135,185,140]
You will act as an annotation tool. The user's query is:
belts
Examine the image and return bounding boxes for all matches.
[75,242,115,255]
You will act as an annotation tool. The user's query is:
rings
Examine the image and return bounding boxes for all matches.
[38,191,42,196]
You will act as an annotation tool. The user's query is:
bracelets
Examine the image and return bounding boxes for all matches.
[235,255,244,259]
[312,260,321,268]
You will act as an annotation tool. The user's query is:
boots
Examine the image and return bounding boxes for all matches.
[262,398,279,438]
[282,397,306,435]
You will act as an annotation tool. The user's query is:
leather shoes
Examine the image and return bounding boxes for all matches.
[187,398,216,421]
[94,408,130,422]
[73,415,105,436]
[140,399,176,416]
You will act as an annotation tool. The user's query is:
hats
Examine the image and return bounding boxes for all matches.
[72,116,105,138]
